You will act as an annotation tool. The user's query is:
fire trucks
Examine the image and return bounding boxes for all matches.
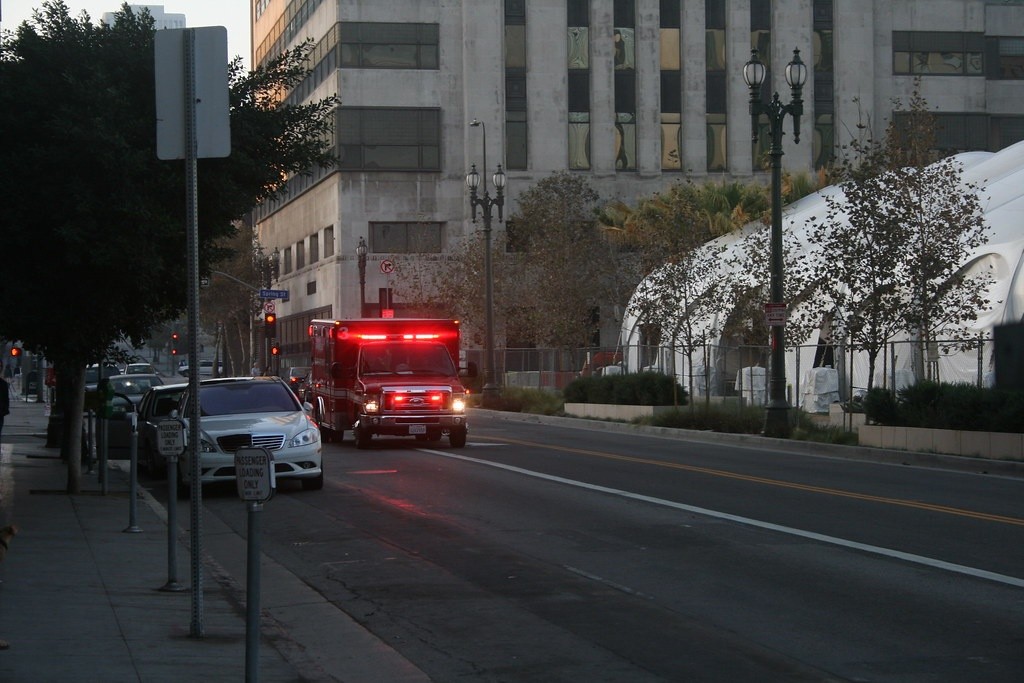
[307,308,478,450]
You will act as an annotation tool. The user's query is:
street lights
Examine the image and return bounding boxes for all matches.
[465,117,507,409]
[355,236,368,317]
[741,44,810,438]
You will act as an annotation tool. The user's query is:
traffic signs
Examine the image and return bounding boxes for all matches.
[762,303,786,327]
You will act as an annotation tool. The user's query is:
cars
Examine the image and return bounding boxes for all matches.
[179,359,223,378]
[98,373,169,426]
[124,381,237,485]
[84,363,122,404]
[171,376,326,493]
[125,363,160,384]
[284,366,313,405]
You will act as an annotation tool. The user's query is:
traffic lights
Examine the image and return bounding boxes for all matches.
[171,333,181,355]
[271,345,280,356]
[11,348,22,357]
[264,312,276,339]
[199,268,211,289]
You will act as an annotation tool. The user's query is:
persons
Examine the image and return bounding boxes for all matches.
[0,377,11,432]
[3,364,20,379]
[252,362,261,377]
